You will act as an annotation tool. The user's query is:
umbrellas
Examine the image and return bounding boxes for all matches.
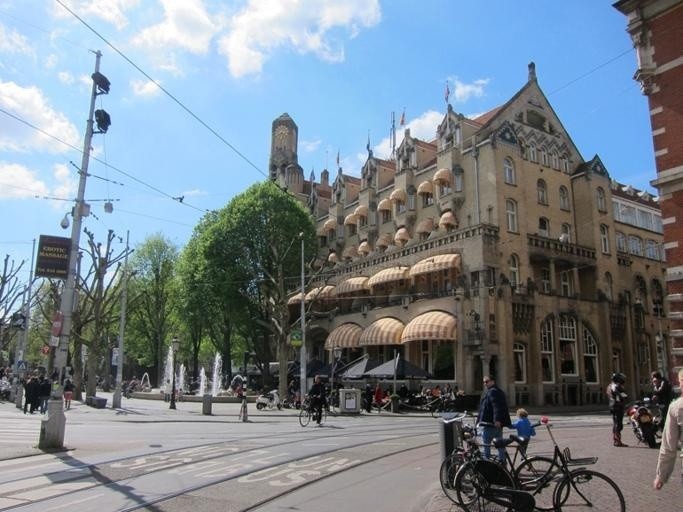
[271,355,433,379]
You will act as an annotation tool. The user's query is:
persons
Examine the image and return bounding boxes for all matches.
[474,373,511,470]
[178,388,183,402]
[175,389,178,402]
[128,375,138,392]
[304,375,325,424]
[430,382,460,413]
[374,383,382,413]
[399,382,407,397]
[18,373,59,416]
[365,383,373,414]
[651,367,683,492]
[163,378,170,402]
[380,390,391,409]
[387,384,392,394]
[605,371,631,447]
[288,378,298,396]
[651,370,674,433]
[139,372,151,390]
[62,379,75,410]
[507,408,536,462]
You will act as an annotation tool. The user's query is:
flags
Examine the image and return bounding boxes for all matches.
[365,135,370,152]
[443,83,451,101]
[399,112,405,126]
[309,170,315,181]
[336,151,340,165]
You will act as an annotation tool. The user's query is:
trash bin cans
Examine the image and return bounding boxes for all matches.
[438,413,465,489]
[339,389,362,415]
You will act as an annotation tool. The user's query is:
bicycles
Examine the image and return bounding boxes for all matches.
[431,409,625,512]
[299,393,327,427]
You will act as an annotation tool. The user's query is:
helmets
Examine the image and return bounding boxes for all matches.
[611,372,626,385]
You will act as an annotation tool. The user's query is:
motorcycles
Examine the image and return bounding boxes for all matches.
[625,397,662,448]
[256,389,283,410]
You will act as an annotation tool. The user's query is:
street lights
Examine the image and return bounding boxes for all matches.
[333,346,343,406]
[170,336,180,410]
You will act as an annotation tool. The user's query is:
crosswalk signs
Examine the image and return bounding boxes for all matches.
[17,360,28,372]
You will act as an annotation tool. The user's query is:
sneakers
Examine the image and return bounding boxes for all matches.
[614,441,627,447]
